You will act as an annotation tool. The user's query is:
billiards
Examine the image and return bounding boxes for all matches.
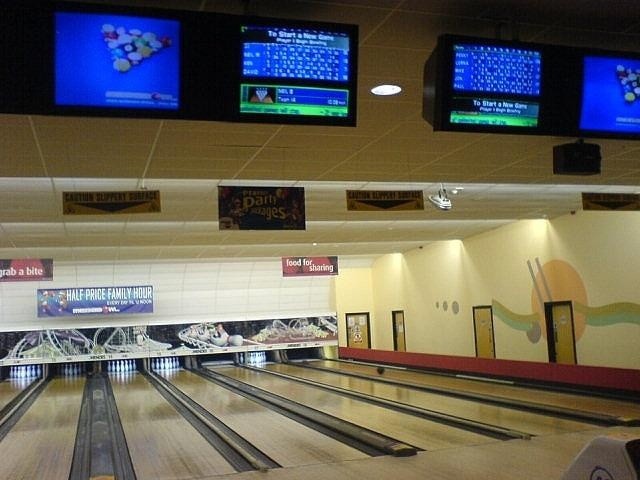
[103,25,172,73]
[616,65,640,101]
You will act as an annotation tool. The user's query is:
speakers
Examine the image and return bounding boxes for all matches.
[421,34,567,136]
[565,46,639,140]
[212,13,358,127]
[552,142,602,178]
[0,0,213,121]
